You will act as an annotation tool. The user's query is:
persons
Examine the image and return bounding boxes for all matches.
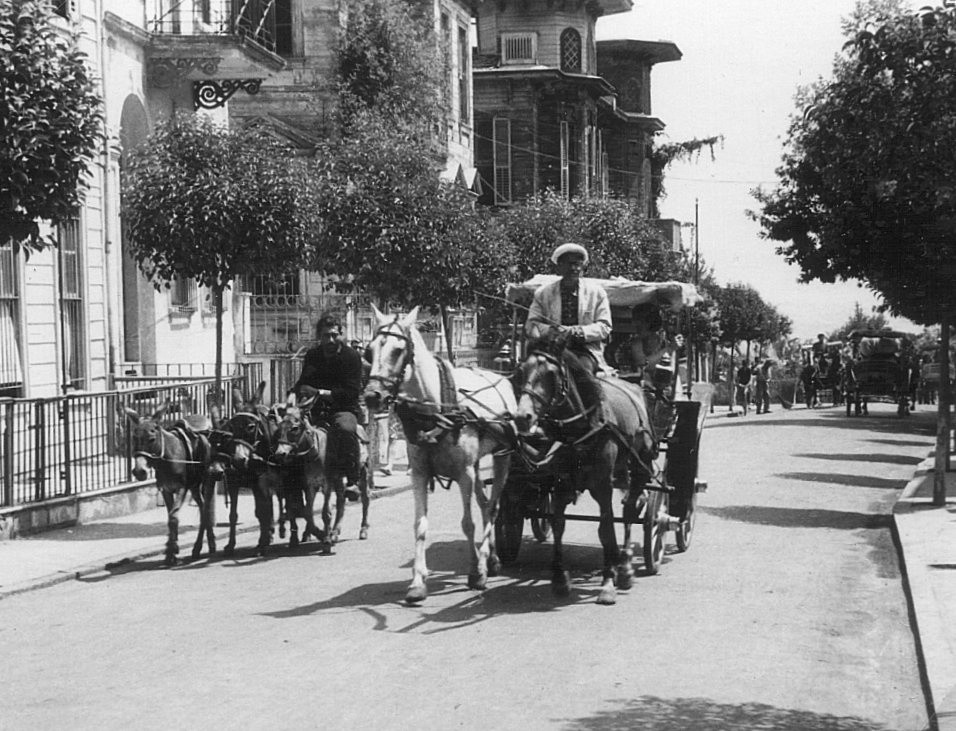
[715,330,955,415]
[523,240,613,440]
[610,302,685,486]
[287,314,391,487]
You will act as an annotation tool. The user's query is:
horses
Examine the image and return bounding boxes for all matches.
[798,364,823,409]
[518,324,659,603]
[362,302,518,603]
[268,391,349,554]
[826,346,846,409]
[122,396,219,566]
[211,380,287,560]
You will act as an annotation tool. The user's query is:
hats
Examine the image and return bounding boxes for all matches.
[551,243,589,266]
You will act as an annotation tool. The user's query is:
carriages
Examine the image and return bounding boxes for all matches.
[797,328,955,418]
[363,267,718,601]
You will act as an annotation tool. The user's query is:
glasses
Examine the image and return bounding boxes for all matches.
[352,346,363,350]
[320,333,340,338]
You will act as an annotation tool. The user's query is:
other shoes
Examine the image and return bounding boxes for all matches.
[343,485,360,502]
